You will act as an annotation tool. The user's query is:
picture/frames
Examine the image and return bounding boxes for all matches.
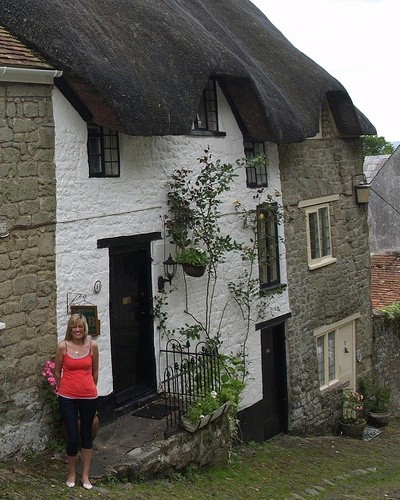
[71,306,99,336]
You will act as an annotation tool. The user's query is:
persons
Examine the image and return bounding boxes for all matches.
[54,314,99,489]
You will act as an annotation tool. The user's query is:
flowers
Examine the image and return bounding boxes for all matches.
[35,359,63,397]
[185,390,221,426]
[343,387,365,423]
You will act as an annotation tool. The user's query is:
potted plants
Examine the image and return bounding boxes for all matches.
[359,374,394,427]
[178,248,209,277]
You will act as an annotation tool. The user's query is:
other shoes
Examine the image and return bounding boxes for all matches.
[81,478,93,490]
[65,481,76,487]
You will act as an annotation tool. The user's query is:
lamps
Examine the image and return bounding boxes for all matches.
[339,173,372,205]
[258,209,267,221]
[158,253,177,293]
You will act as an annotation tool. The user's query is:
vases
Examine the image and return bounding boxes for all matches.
[181,401,229,432]
[77,412,100,445]
[339,419,367,438]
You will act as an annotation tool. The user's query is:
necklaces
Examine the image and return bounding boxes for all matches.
[75,351,79,354]
